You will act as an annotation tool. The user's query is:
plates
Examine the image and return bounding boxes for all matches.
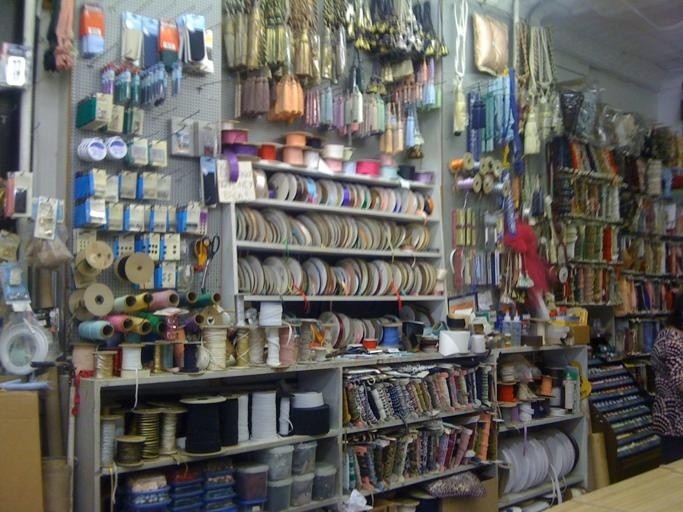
[233,252,438,300]
[315,308,432,350]
[271,172,433,215]
[235,204,433,252]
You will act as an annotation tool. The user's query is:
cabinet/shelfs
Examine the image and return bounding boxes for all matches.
[71,340,593,512]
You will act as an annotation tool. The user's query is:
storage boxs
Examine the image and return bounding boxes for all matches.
[293,440,317,473]
[233,461,269,499]
[206,153,446,355]
[239,499,268,511]
[202,458,236,511]
[248,446,294,482]
[167,464,204,512]
[122,472,170,512]
[314,460,336,499]
[266,479,290,510]
[291,474,314,505]
